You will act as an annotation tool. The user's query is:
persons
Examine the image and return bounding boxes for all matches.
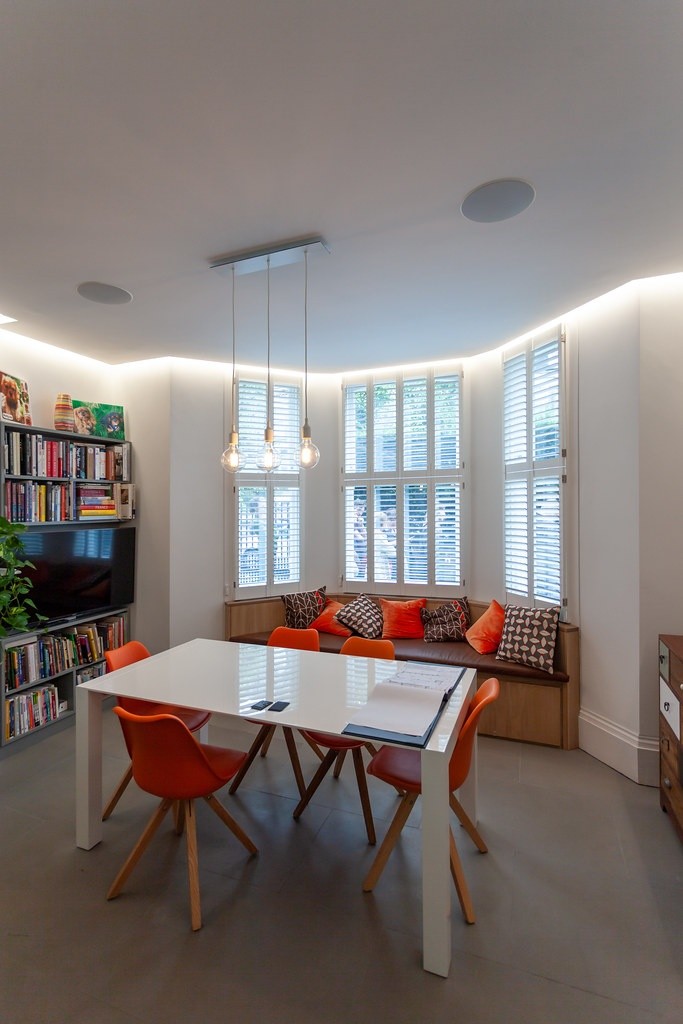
[352,499,397,583]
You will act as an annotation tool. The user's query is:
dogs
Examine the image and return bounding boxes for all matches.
[100,412,123,433]
[1,375,21,422]
[73,407,97,435]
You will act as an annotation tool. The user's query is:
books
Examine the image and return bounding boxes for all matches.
[5,616,124,742]
[341,660,467,749]
[4,431,136,522]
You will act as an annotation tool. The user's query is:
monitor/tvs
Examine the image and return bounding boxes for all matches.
[0,527,136,630]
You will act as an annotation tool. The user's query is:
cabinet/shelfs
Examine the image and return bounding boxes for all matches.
[658,634,683,843]
[0,420,131,526]
[0,607,130,746]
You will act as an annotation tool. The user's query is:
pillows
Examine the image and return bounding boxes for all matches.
[466,599,505,654]
[307,596,353,637]
[334,593,384,638]
[495,604,561,675]
[281,586,326,629]
[379,597,427,639]
[421,595,472,643]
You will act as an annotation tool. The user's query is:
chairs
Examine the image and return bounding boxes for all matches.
[108,707,258,931]
[362,678,499,924]
[228,628,325,795]
[293,636,394,845]
[101,641,211,821]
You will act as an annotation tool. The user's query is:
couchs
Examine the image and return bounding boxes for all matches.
[226,593,579,751]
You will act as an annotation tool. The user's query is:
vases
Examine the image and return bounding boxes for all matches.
[54,394,74,430]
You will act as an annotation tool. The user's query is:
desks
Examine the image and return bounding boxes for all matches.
[75,637,477,977]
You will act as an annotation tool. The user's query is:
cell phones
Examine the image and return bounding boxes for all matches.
[251,701,273,710]
[268,702,290,712]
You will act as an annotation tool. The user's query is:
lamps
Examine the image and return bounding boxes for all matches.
[210,238,333,473]
